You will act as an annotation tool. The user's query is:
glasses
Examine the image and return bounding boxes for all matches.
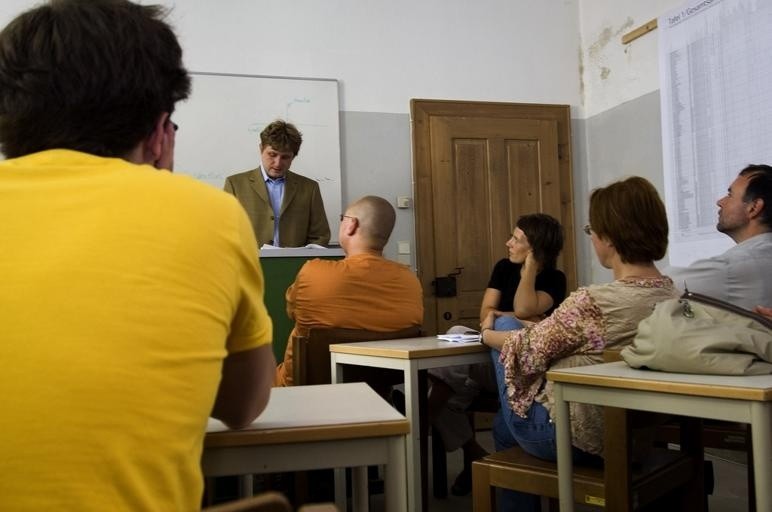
[339,213,353,222]
[581,224,604,237]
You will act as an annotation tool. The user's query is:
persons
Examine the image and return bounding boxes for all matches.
[275,194,425,390]
[478,174,682,511]
[223,117,332,249]
[427,212,569,499]
[683,162,772,328]
[0,0,277,512]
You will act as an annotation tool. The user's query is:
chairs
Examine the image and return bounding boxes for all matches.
[294,327,420,417]
[473,351,704,511]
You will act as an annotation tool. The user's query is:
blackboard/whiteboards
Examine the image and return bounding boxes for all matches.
[170,70,344,245]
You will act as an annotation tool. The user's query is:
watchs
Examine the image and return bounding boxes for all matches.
[478,327,493,347]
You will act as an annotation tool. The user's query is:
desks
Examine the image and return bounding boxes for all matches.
[331,334,493,511]
[547,360,772,511]
[200,382,410,511]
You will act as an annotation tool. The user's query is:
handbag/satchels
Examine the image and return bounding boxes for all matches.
[618,291,772,376]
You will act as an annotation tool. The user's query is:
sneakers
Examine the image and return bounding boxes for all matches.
[450,452,490,497]
[391,389,405,415]
